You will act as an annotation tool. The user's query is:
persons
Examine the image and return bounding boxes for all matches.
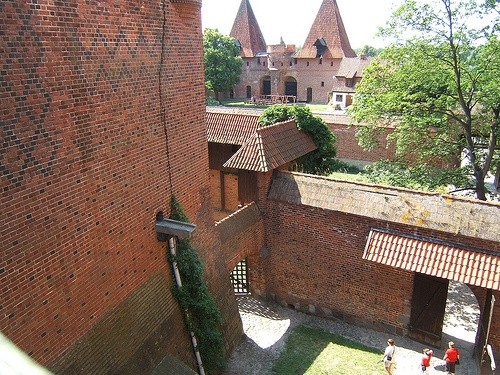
[249,94,258,108]
[443,341,460,375]
[376,338,396,375]
[419,348,434,375]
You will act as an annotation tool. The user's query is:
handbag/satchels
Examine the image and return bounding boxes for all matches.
[387,356,392,361]
[422,365,426,371]
